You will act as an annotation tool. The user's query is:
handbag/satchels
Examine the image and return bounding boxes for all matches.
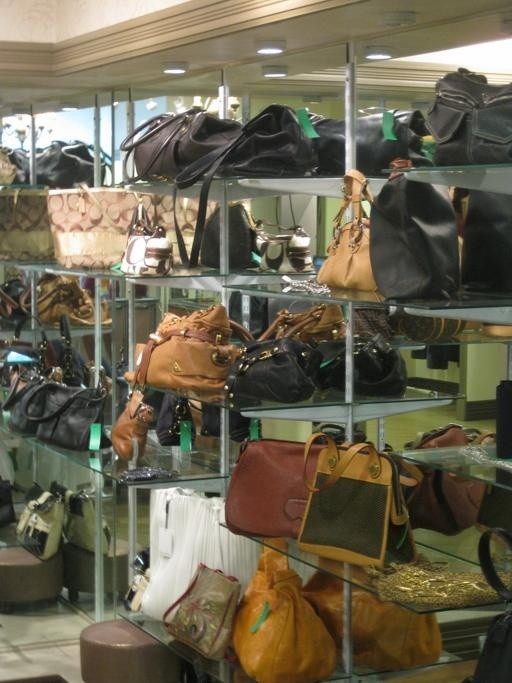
[0,67,512,304]
[224,426,486,570]
[123,549,150,612]
[50,481,112,554]
[163,538,443,679]
[475,528,511,681]
[0,266,467,463]
[16,484,64,561]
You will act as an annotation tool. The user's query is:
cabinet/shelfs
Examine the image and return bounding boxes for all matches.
[1,37,512,683]
